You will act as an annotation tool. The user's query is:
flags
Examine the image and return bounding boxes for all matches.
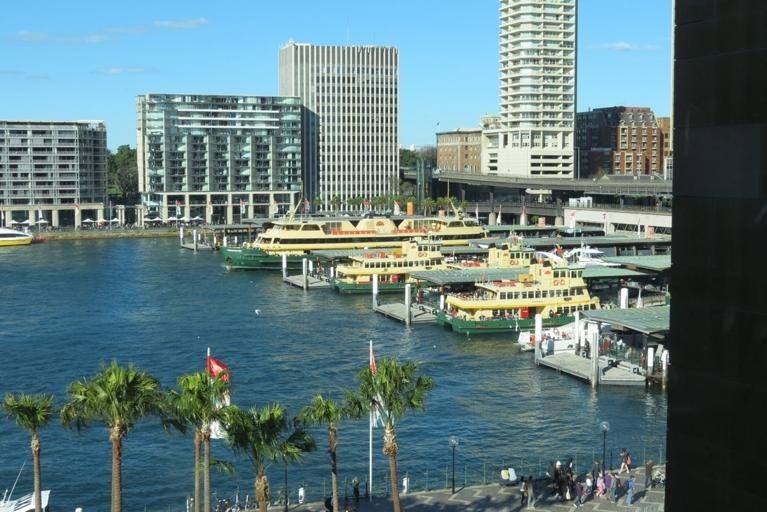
[206,355,231,439]
[370,351,395,428]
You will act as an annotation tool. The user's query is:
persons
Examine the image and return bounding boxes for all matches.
[324,492,333,512]
[414,278,647,366]
[498,447,654,509]
[402,476,409,494]
[353,479,360,503]
[298,485,305,504]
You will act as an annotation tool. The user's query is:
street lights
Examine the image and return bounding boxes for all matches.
[447,435,461,495]
[599,420,610,477]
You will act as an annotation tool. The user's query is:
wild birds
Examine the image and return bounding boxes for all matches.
[254,308,262,317]
